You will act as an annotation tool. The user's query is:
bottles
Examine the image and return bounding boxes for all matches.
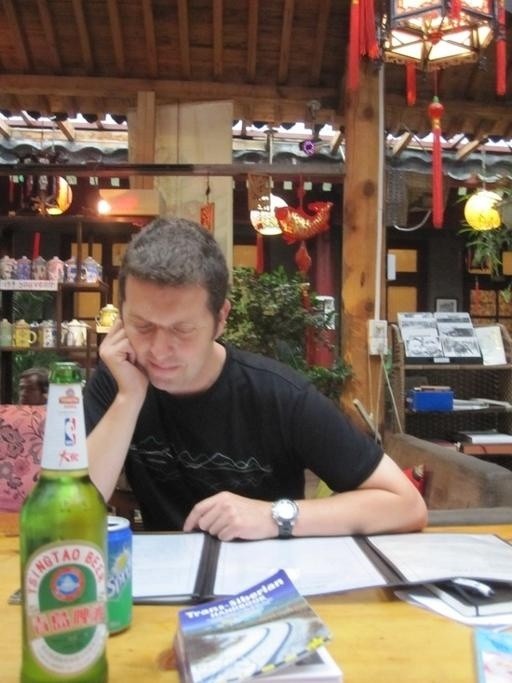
[0,254,120,346]
[21,363,109,678]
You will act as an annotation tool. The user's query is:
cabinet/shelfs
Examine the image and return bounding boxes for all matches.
[0,212,141,403]
[387,322,511,461]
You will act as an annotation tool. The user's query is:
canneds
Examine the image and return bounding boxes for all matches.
[106,515,132,637]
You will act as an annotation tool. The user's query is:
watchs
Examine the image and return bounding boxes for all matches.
[268,495,301,540]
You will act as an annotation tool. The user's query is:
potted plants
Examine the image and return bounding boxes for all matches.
[216,262,357,497]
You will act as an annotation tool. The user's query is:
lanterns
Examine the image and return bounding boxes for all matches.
[346,0,508,230]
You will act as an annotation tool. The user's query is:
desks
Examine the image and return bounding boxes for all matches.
[2,524,511,681]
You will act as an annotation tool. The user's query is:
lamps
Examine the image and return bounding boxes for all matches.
[464,142,504,232]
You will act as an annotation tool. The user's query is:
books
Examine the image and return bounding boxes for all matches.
[472,624,511,683]
[4,528,511,631]
[396,308,512,447]
[170,568,344,683]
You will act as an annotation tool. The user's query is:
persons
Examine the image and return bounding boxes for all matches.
[82,213,430,542]
[15,365,51,404]
